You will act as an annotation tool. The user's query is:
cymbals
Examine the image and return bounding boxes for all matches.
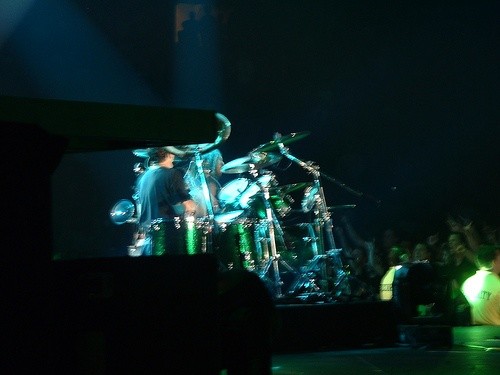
[249,181,310,200]
[221,152,282,174]
[250,130,312,154]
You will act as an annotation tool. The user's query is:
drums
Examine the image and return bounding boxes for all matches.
[149,215,209,254]
[254,223,319,293]
[210,210,259,271]
[217,178,267,218]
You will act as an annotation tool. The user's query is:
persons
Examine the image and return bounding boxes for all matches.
[137,147,204,227]
[183,149,224,215]
[334,215,500,326]
